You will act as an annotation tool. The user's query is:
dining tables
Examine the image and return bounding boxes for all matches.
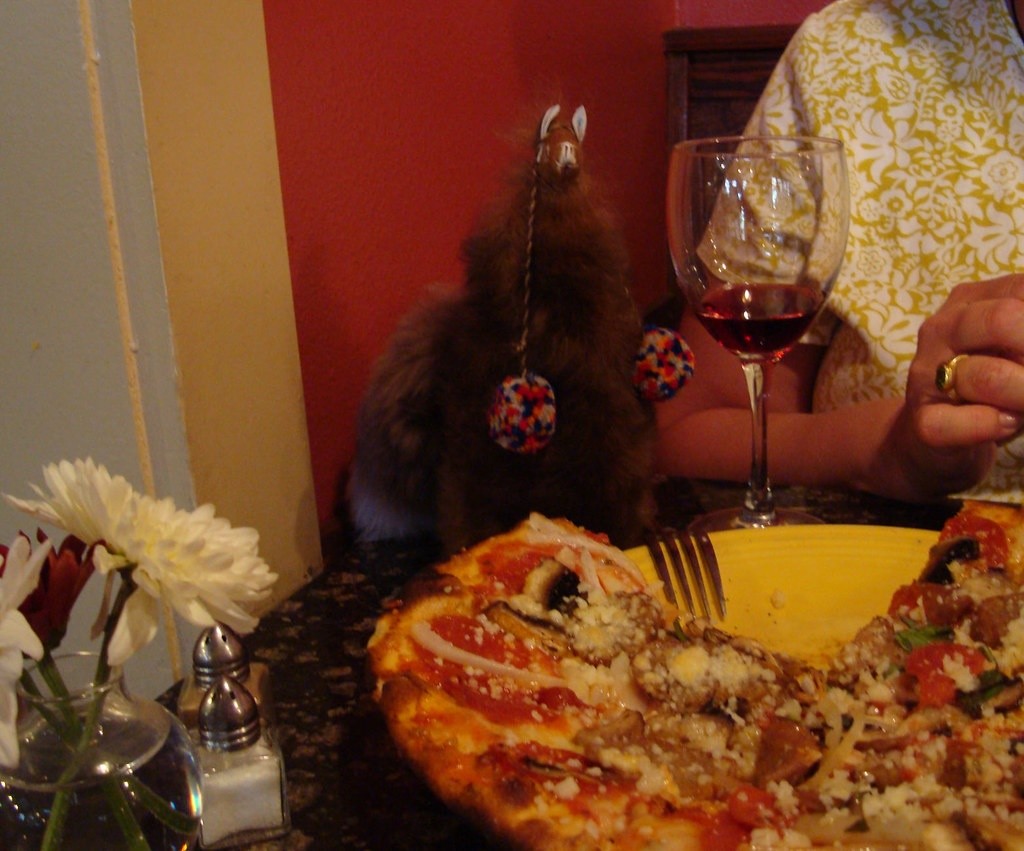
[157,480,973,850]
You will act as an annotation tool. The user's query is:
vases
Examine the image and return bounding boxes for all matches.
[0,653,203,851]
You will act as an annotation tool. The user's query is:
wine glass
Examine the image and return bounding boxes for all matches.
[667,133,851,539]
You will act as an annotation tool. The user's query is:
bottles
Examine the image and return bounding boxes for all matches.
[178,625,272,747]
[180,681,287,850]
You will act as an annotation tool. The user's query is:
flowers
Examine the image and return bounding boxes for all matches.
[0,455,277,851]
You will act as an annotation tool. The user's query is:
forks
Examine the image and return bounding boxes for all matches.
[632,523,729,619]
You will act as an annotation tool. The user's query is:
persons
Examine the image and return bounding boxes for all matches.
[654,0,1024,505]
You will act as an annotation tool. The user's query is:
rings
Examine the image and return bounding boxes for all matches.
[935,354,970,405]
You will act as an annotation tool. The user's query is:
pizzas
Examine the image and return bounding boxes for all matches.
[369,499,1024,851]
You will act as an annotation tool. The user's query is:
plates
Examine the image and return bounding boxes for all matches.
[619,525,943,672]
